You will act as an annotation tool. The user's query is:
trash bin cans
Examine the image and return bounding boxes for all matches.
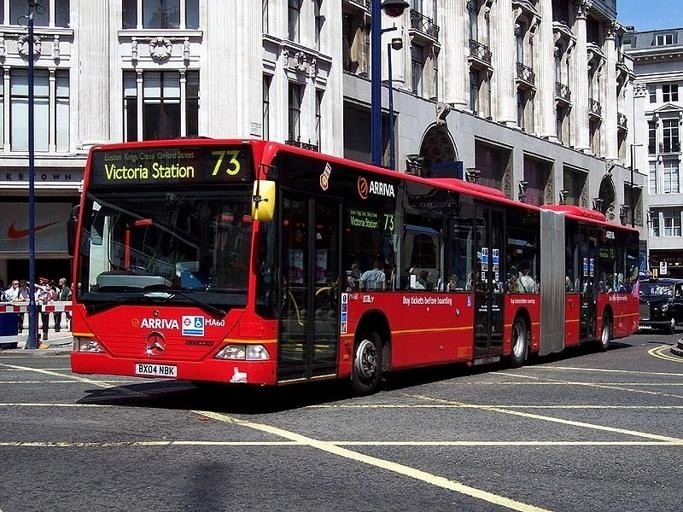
[0,313,18,349]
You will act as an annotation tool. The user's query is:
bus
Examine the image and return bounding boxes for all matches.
[67,138,640,390]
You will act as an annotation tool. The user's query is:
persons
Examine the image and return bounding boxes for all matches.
[345,252,640,294]
[0,277,74,340]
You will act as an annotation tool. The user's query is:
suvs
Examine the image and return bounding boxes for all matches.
[640,277,682,334]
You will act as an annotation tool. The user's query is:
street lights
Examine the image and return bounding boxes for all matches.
[370,0,411,170]
[630,143,643,227]
